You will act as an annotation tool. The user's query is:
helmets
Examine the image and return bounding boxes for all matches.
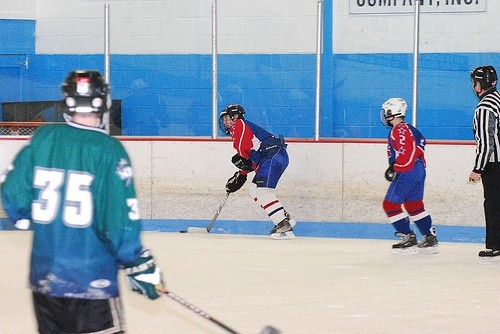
[61,70,112,115]
[382,97,407,117]
[219,104,245,120]
[471,65,497,90]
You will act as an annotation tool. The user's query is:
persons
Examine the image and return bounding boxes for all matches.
[468,65,500,257]
[219,104,297,239]
[380,97,440,255]
[0,70,167,334]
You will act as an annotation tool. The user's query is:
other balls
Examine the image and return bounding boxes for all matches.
[180,230,187,233]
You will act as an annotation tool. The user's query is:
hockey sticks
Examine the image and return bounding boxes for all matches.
[158,286,282,334]
[187,169,241,234]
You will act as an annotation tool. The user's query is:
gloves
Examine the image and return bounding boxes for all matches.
[225,171,247,192]
[231,153,252,170]
[124,250,166,300]
[385,162,399,181]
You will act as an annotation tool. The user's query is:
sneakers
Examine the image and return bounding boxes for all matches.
[392,229,418,254]
[271,209,296,240]
[479,248,500,264]
[418,227,439,254]
